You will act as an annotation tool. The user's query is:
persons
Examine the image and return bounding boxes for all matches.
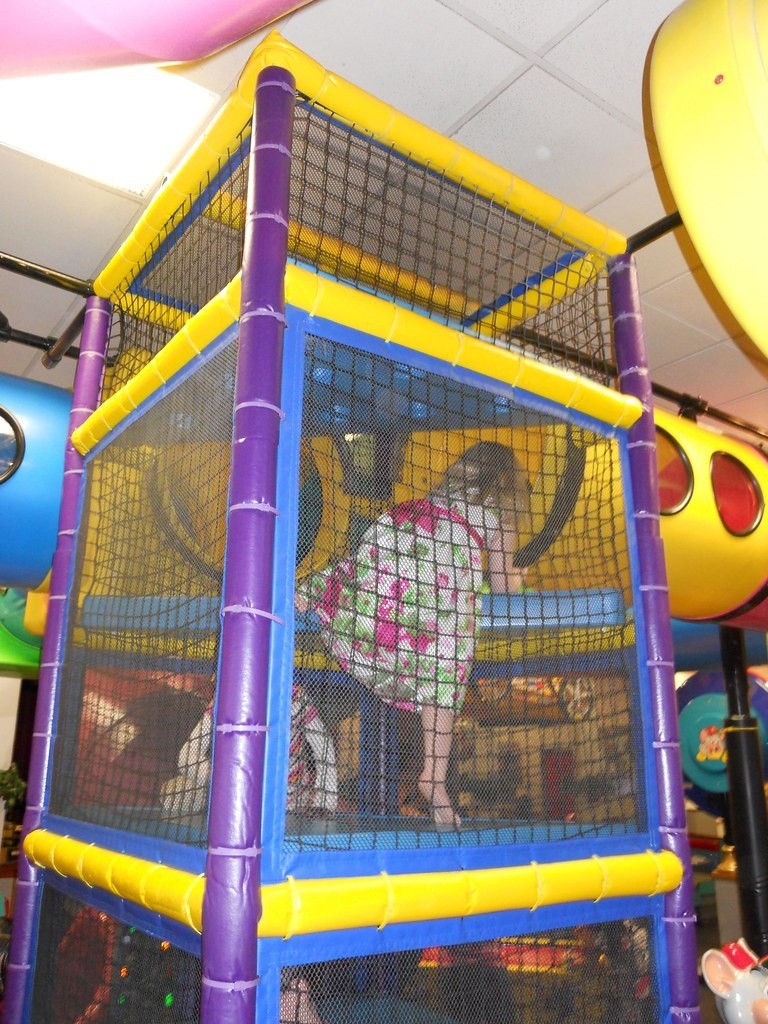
[160,679,338,822]
[292,442,531,825]
